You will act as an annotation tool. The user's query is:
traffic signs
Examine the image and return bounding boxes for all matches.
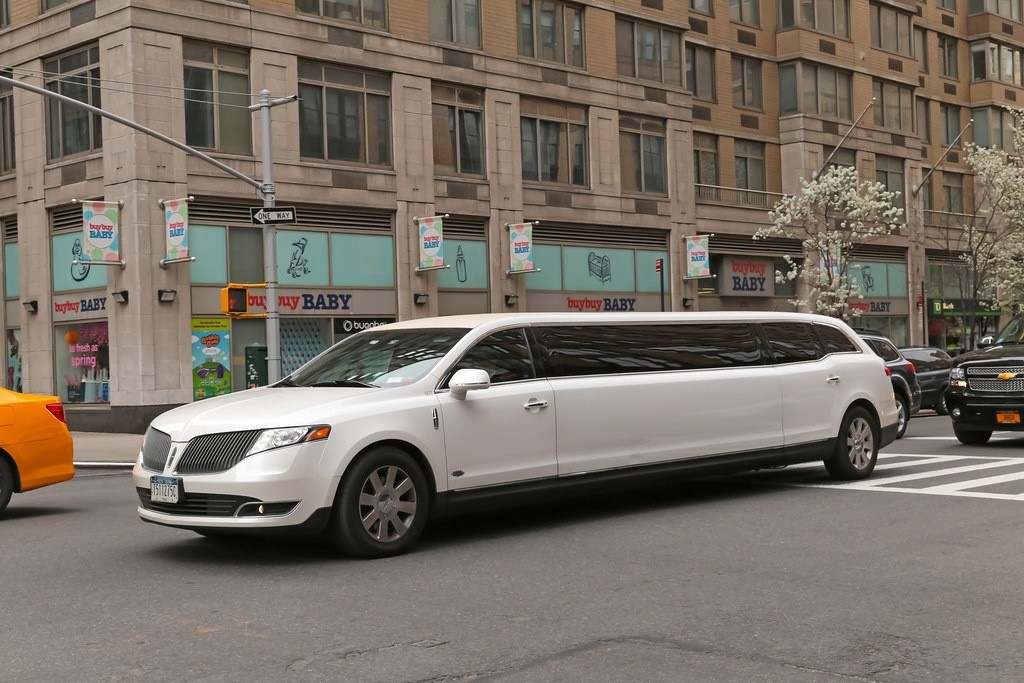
[250,205,296,225]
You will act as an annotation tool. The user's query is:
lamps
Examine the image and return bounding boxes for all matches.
[109,290,130,306]
[681,296,697,307]
[412,292,432,304]
[505,294,521,305]
[21,297,38,315]
[159,288,180,303]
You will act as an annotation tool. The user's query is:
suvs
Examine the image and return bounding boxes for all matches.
[896,346,955,415]
[857,334,922,440]
[947,310,1024,446]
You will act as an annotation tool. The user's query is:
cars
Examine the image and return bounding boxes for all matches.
[131,310,899,559]
[0,387,76,510]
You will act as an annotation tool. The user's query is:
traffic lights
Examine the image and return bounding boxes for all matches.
[220,286,250,317]
